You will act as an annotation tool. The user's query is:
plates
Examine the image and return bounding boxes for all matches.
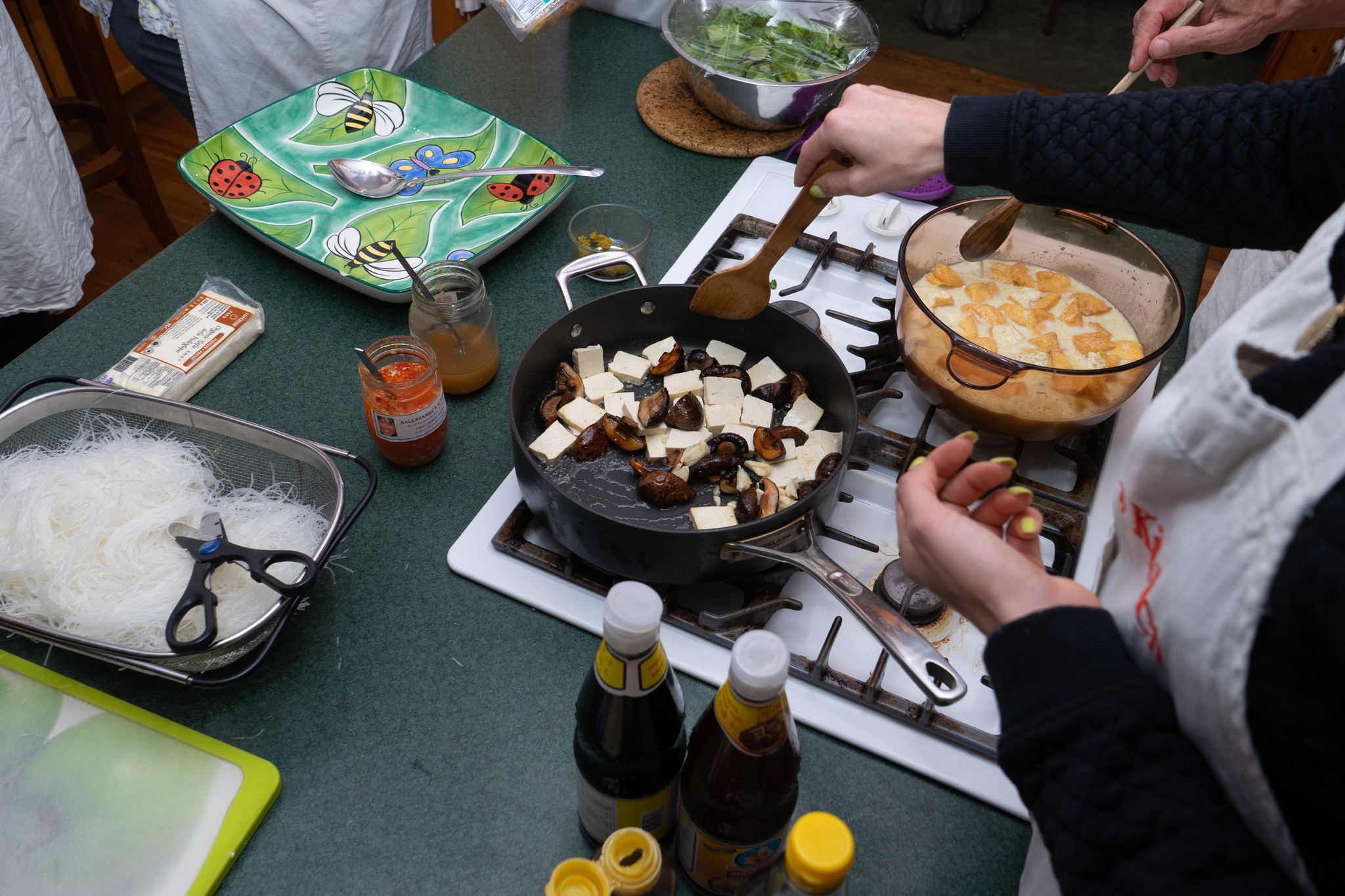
[177,67,577,303]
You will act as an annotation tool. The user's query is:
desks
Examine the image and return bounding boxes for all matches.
[0,0,1213,896]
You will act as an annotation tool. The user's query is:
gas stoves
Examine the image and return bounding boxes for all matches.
[448,154,1172,822]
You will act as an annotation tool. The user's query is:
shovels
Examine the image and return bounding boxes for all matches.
[690,161,847,321]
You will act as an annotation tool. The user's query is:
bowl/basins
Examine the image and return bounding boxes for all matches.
[568,204,653,282]
[660,0,879,131]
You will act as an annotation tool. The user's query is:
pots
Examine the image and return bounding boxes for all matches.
[897,196,1187,440]
[508,251,968,706]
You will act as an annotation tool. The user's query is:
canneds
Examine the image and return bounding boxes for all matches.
[408,260,500,395]
[358,335,448,469]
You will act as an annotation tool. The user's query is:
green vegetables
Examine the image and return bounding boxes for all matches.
[680,5,867,85]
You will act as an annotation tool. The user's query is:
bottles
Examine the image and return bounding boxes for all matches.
[408,260,499,394]
[672,628,801,896]
[732,811,854,896]
[574,580,687,850]
[544,826,675,896]
[358,335,447,467]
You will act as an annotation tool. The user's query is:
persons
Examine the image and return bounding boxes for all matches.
[1128,0,1345,364]
[794,73,1345,896]
[0,0,95,366]
[81,0,436,210]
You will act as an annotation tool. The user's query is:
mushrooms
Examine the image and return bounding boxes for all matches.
[537,341,844,522]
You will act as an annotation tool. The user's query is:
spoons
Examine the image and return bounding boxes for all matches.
[327,158,604,199]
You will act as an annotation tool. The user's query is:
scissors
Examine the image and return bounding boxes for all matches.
[164,513,318,655]
